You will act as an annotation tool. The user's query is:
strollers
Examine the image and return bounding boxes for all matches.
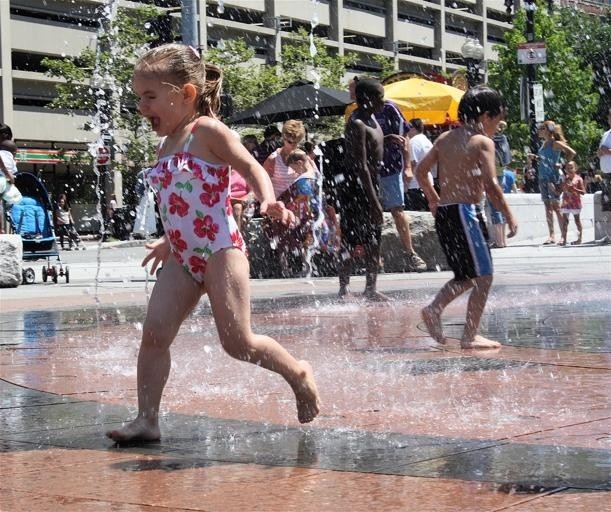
[62,223,86,251]
[4,172,69,283]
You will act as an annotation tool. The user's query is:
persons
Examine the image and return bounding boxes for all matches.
[225,108,437,278]
[489,121,611,248]
[105,41,321,449]
[413,85,517,350]
[0,123,18,235]
[346,79,427,272]
[106,194,118,224]
[339,77,408,304]
[54,198,86,250]
[54,193,75,250]
[0,139,23,223]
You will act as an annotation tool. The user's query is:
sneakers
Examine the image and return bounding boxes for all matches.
[402,252,428,273]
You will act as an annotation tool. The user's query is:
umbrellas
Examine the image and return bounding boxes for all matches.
[220,77,356,141]
[343,74,466,125]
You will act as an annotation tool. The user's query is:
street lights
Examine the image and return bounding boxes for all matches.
[461,37,485,86]
